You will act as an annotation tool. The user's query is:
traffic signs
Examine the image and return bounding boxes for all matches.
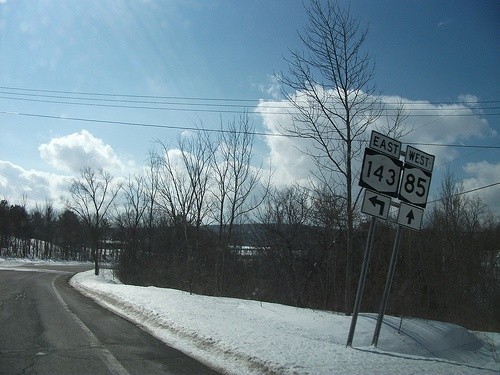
[357,128,436,230]
